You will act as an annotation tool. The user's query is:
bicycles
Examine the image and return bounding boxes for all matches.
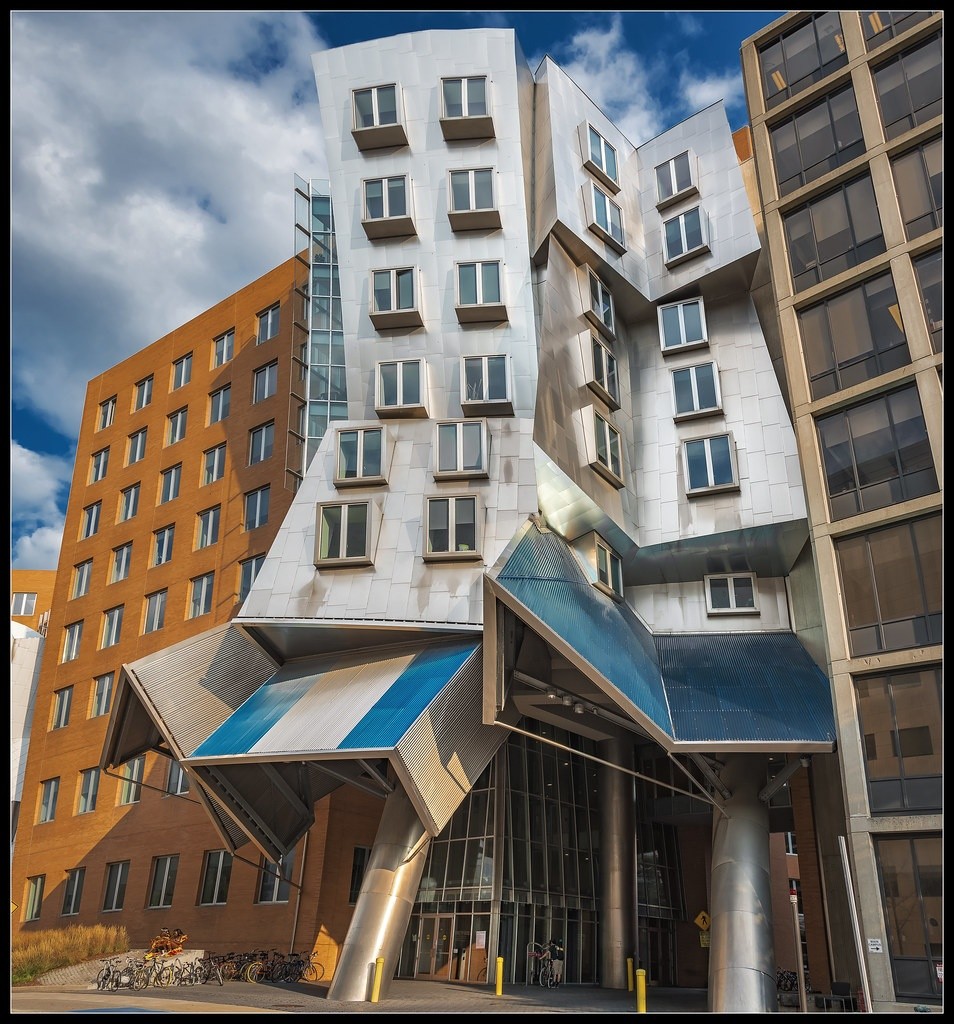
[477,957,489,981]
[96,946,326,994]
[776,967,810,993]
[537,957,558,989]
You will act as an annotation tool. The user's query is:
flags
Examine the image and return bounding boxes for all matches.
[528,949,542,958]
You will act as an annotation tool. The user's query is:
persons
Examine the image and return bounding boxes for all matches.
[144,926,188,961]
[539,940,563,989]
